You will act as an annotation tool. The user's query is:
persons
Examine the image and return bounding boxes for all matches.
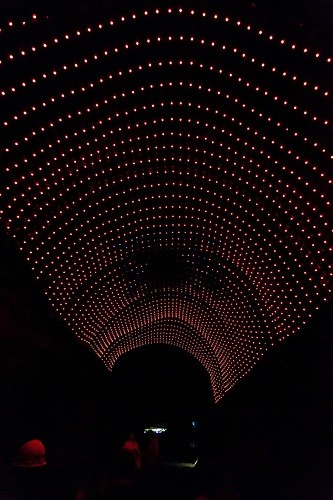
[2,421,244,499]
